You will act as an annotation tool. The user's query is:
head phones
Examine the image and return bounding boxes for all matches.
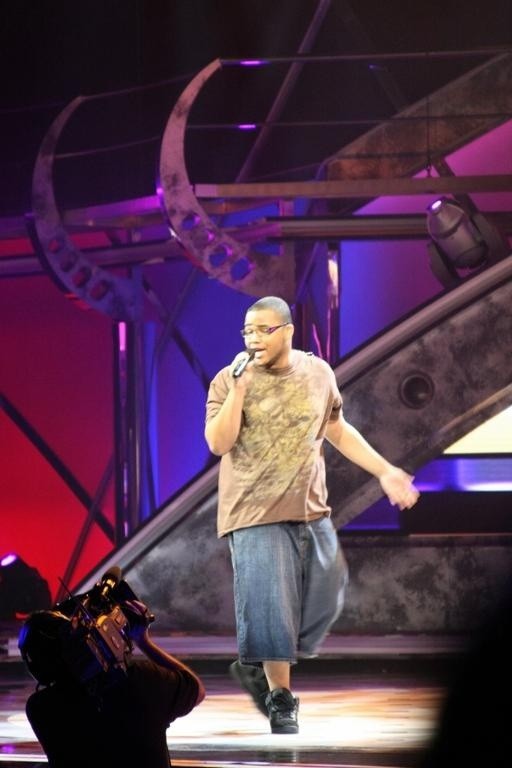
[21,615,82,686]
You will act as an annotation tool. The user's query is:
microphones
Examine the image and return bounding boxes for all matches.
[232,348,256,378]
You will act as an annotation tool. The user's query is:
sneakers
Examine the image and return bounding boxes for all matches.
[228,658,271,719]
[263,685,301,736]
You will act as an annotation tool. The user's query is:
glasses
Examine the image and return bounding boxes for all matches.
[240,322,291,338]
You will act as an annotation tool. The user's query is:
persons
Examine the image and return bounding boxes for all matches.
[203,295,422,734]
[18,611,205,768]
